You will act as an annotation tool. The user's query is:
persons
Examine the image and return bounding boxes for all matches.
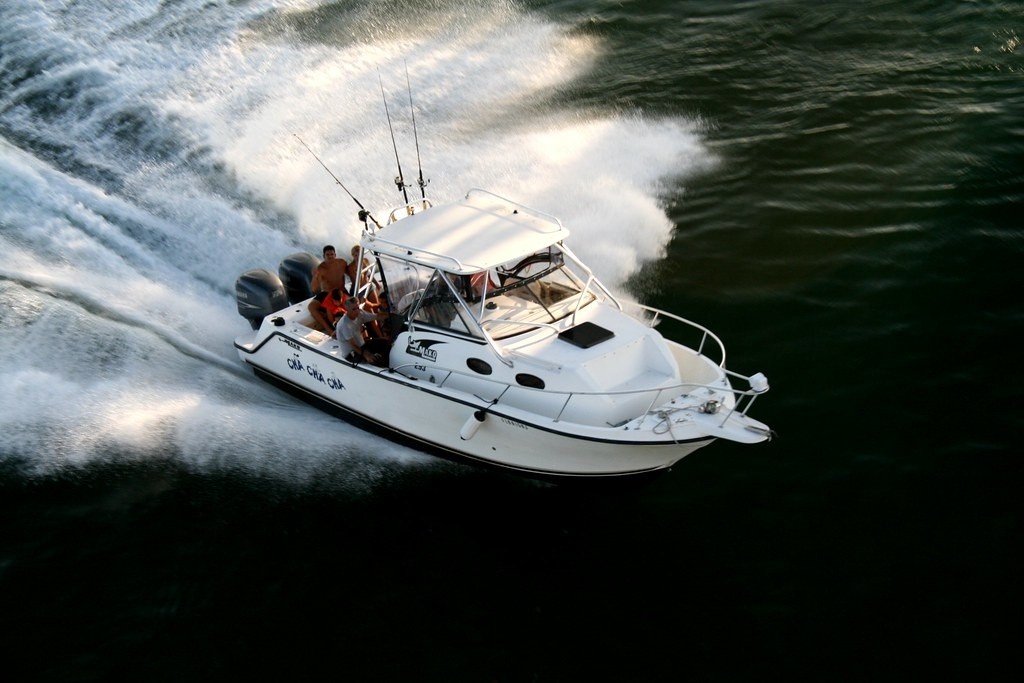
[308,245,409,368]
[423,271,463,329]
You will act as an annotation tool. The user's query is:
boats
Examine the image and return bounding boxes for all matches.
[228,68,778,484]
